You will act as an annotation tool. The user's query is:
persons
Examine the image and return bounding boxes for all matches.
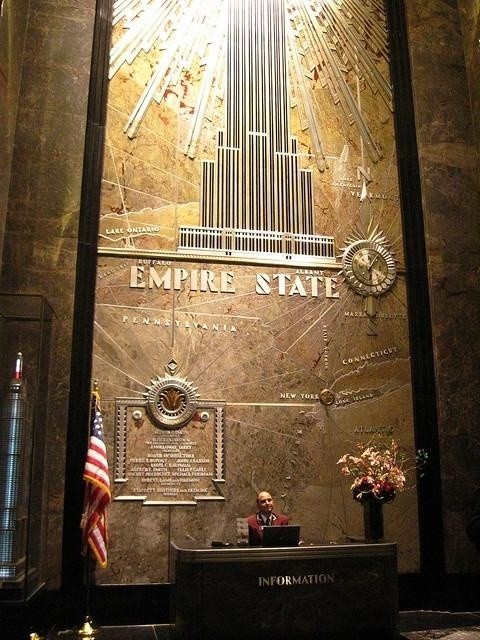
[246,491,289,547]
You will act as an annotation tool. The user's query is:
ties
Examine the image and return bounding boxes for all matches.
[266,518,270,525]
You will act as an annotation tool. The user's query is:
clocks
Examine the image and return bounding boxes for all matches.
[342,240,396,299]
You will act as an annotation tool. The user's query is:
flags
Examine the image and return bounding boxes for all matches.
[81,392,112,570]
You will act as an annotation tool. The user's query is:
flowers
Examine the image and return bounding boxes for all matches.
[335,430,429,507]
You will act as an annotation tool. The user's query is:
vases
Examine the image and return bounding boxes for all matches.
[360,495,386,542]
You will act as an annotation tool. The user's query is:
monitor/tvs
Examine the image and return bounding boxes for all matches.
[263,526,302,547]
